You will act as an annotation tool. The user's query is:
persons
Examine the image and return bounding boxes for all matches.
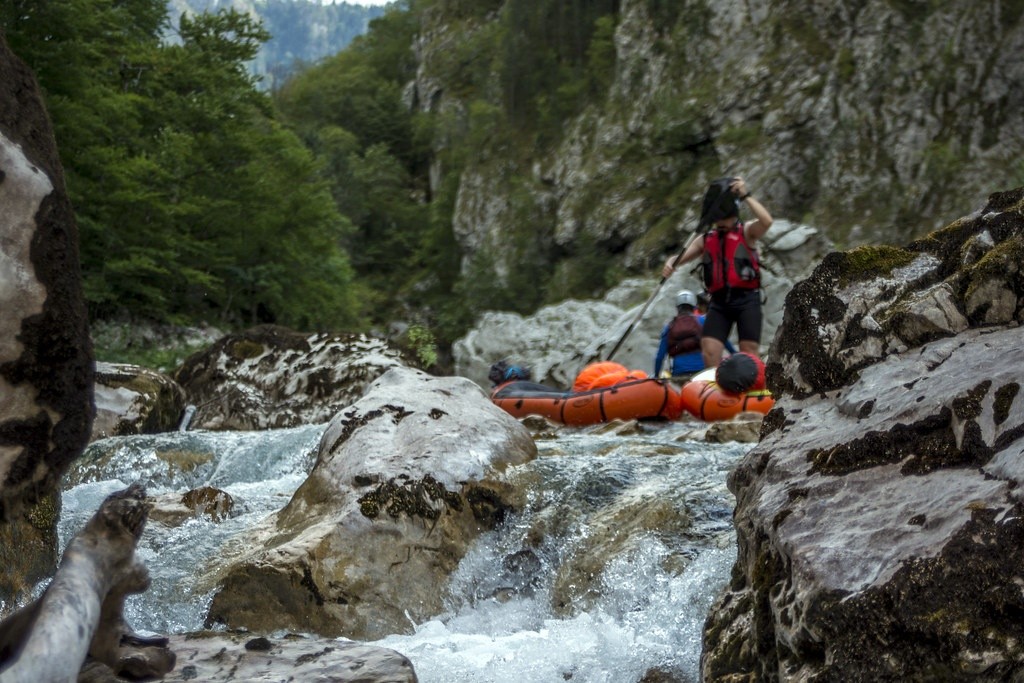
[654,290,739,386]
[663,176,774,366]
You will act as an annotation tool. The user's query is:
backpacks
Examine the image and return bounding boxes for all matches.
[664,315,704,357]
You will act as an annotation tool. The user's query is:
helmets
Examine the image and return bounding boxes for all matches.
[674,289,698,308]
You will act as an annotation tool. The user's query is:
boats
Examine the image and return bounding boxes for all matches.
[659,352,776,422]
[488,377,683,426]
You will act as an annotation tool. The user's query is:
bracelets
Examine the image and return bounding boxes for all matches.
[738,191,753,201]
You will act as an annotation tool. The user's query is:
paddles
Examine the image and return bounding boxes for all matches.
[603,220,705,366]
